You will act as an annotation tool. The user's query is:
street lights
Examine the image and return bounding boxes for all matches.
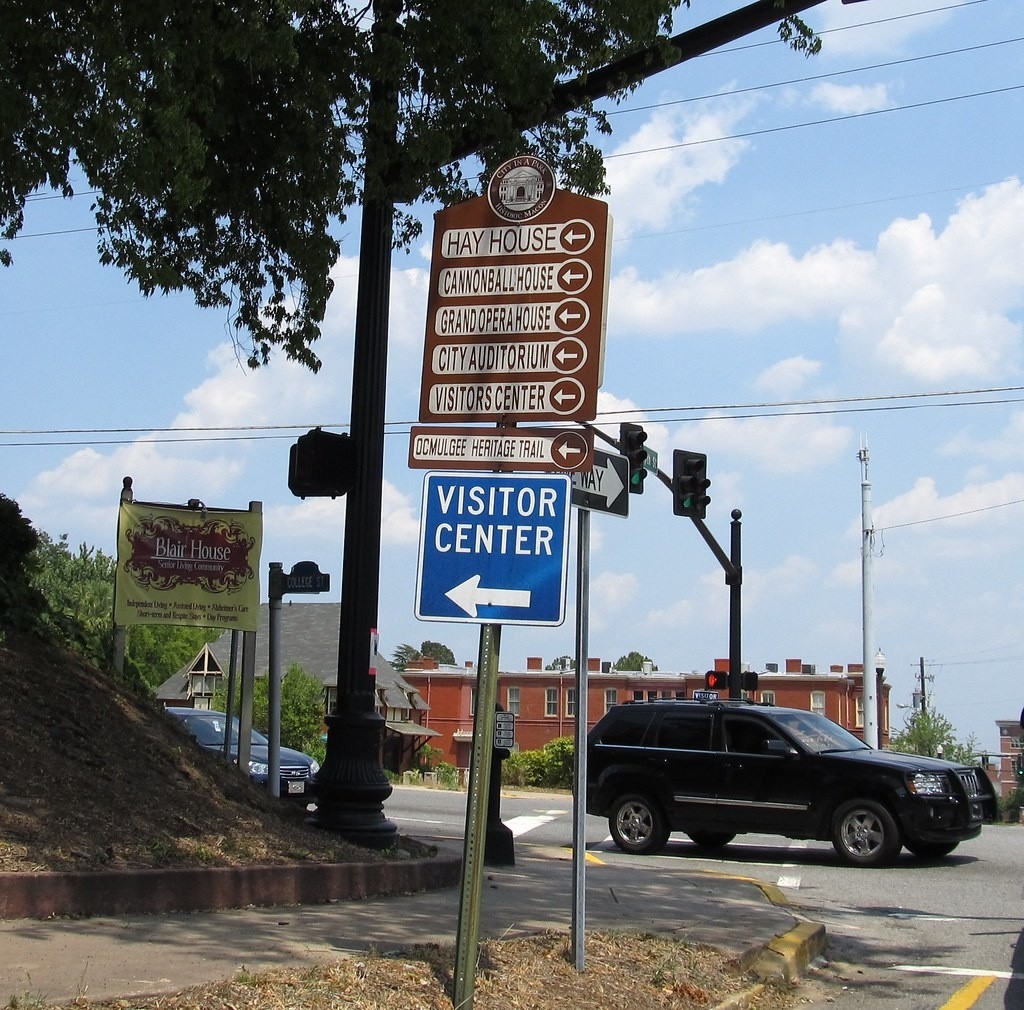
[875,647,887,750]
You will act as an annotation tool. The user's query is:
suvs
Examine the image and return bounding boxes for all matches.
[571,696,997,868]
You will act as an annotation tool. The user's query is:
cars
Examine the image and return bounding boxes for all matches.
[165,706,318,807]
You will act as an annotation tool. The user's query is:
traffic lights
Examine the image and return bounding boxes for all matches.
[741,672,759,691]
[1016,755,1024,776]
[704,671,727,691]
[616,419,650,497]
[669,448,713,520]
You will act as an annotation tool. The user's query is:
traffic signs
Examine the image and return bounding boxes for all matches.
[543,447,631,518]
[404,154,596,469]
[413,470,567,631]
